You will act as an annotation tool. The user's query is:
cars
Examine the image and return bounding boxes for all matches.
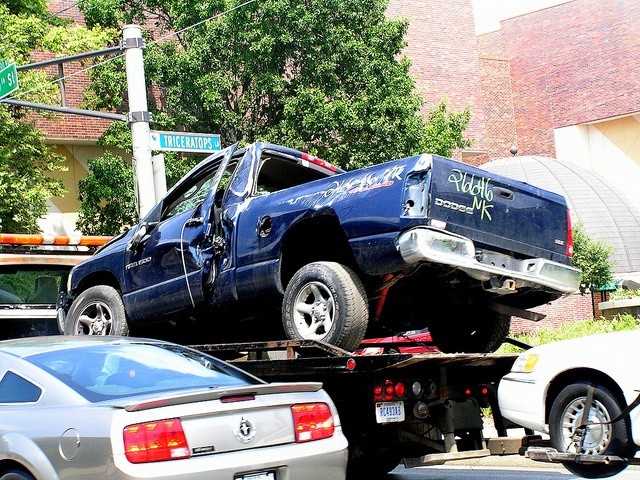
[496,327,640,480]
[1,334,348,480]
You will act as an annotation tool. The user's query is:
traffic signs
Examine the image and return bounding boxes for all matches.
[0,62,21,101]
[152,130,222,153]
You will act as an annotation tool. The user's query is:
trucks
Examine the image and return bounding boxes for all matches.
[0,230,527,479]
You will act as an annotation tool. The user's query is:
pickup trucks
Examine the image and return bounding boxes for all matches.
[62,139,584,357]
